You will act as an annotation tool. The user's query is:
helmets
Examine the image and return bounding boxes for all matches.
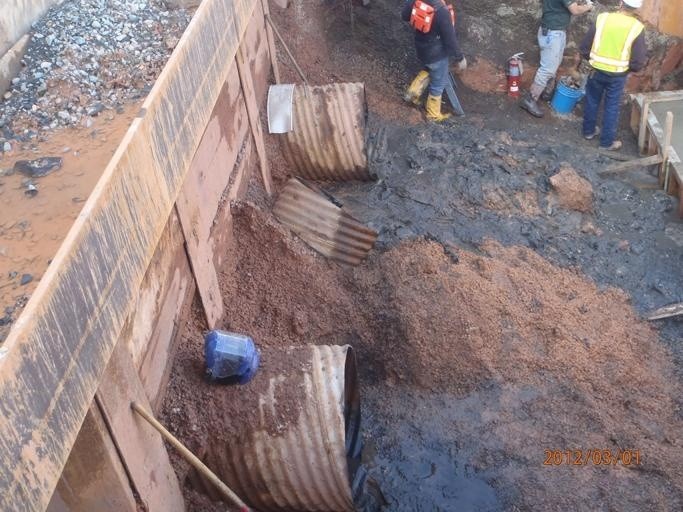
[623,0,643,8]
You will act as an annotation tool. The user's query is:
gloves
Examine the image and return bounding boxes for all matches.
[457,57,467,72]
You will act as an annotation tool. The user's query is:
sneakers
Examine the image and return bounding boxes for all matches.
[598,141,623,152]
[585,126,601,140]
[519,92,544,119]
[542,86,556,101]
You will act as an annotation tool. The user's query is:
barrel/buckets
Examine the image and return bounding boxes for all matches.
[549,77,582,114]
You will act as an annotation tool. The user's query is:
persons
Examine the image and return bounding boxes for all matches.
[515,0,593,118]
[576,0,647,151]
[401,0,467,122]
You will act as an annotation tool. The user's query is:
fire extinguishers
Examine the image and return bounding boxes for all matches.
[506,51,524,96]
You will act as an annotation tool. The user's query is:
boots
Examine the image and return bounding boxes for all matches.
[425,94,453,122]
[402,70,430,105]
